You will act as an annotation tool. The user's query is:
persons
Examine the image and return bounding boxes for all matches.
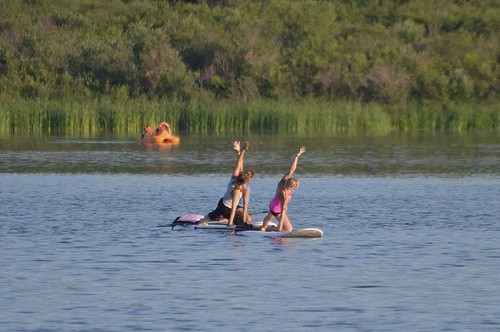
[144,124,167,137]
[214,141,255,222]
[197,141,249,228]
[261,146,306,233]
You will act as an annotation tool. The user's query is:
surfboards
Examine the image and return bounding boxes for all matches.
[230,229,323,237]
[194,214,277,230]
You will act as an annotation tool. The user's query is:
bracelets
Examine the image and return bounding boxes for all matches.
[237,151,240,153]
[296,155,298,156]
